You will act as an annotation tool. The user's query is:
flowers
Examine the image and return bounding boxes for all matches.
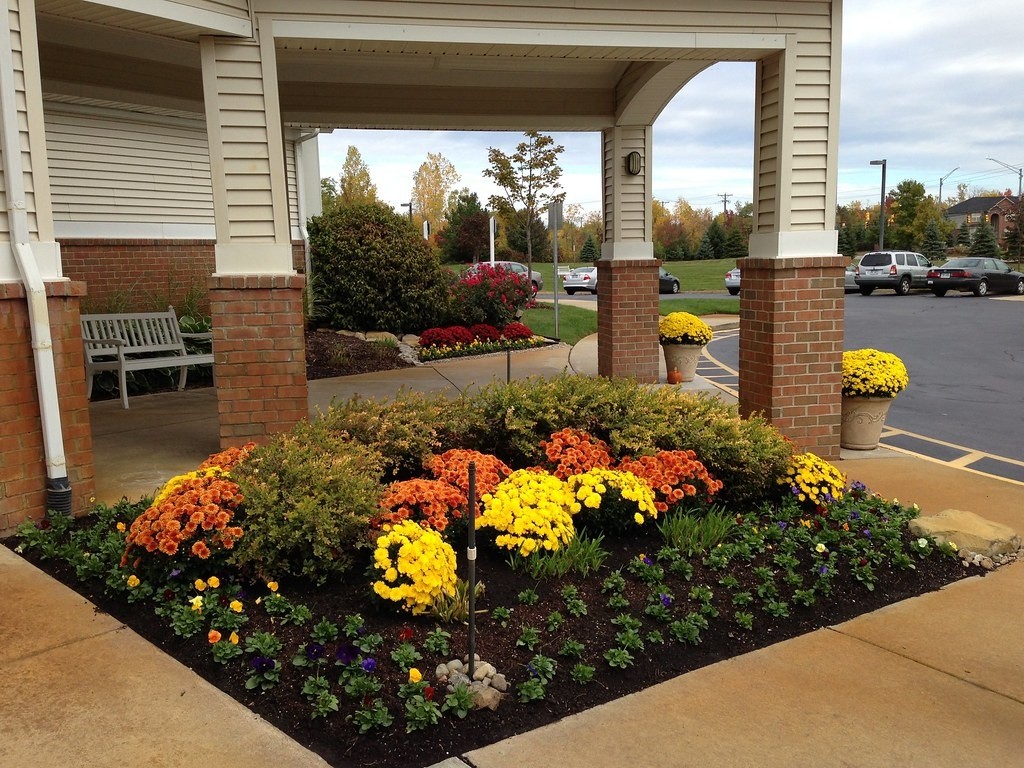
[842,347,909,398]
[659,312,713,346]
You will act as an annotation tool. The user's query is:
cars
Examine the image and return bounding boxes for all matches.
[725,265,742,295]
[658,266,681,294]
[926,256,1024,298]
[845,263,863,293]
[466,259,544,299]
[562,266,597,295]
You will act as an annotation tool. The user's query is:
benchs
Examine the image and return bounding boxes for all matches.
[74,304,216,408]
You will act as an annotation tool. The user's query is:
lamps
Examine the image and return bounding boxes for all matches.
[626,150,643,175]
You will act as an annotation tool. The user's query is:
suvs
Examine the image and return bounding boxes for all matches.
[854,250,941,296]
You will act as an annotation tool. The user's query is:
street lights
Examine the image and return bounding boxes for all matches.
[939,166,959,208]
[401,203,413,223]
[870,159,887,251]
[987,156,1023,202]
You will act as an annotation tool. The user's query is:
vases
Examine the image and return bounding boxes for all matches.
[842,395,891,451]
[660,342,704,382]
